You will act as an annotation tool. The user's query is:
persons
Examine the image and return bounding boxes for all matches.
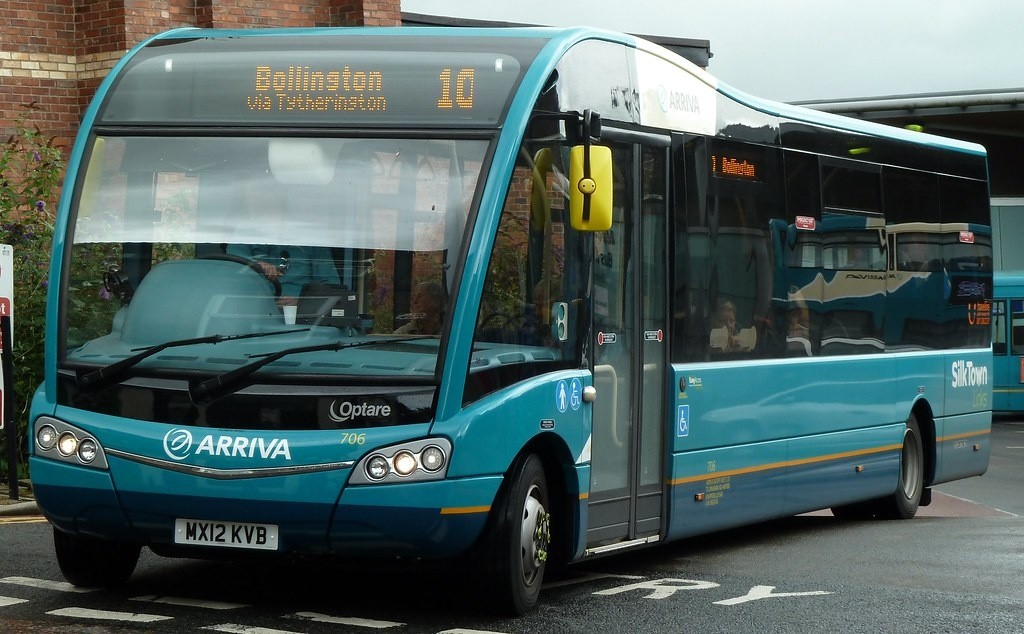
[522,280,565,347]
[704,297,758,361]
[392,281,447,339]
[847,248,872,270]
[225,187,341,313]
[786,286,810,353]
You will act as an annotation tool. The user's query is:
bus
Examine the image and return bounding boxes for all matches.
[765,216,992,350]
[25,25,997,621]
[987,197,1024,411]
